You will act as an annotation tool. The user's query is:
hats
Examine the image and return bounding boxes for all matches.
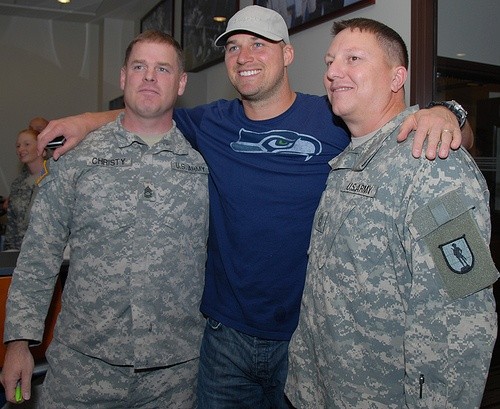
[214,5,291,50]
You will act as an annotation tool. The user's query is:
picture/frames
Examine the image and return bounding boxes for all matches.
[252,0,376,35]
[140,0,174,40]
[180,0,240,72]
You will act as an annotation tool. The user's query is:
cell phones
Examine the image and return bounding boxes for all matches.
[45,135,66,149]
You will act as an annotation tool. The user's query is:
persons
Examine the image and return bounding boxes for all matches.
[283,16,499,409]
[3,129,46,267]
[28,117,48,132]
[36,4,476,409]
[0,30,210,408]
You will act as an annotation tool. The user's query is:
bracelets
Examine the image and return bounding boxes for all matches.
[424,99,467,129]
